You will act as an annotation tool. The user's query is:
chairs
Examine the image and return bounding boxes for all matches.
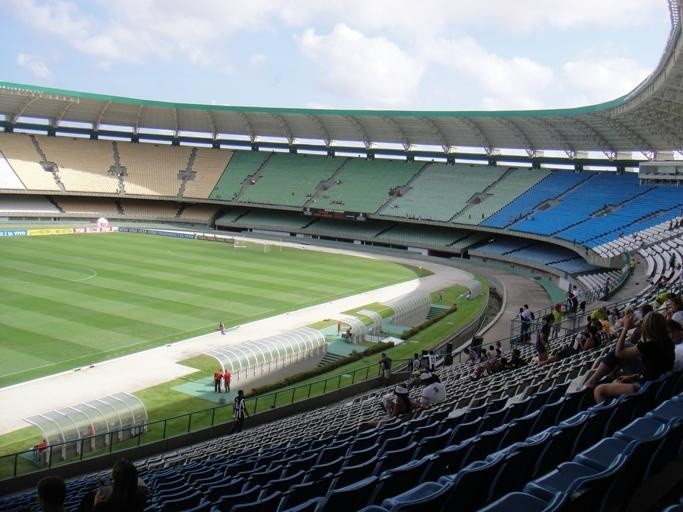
[0,214,683,512]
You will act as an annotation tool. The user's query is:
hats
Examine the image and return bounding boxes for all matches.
[412,371,432,381]
[395,386,408,394]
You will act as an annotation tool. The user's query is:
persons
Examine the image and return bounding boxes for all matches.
[337,321,341,335]
[223,369,233,393]
[630,258,636,273]
[345,326,352,339]
[36,438,47,460]
[77,458,150,512]
[36,476,66,512]
[651,253,680,291]
[464,341,526,380]
[514,285,682,404]
[82,424,93,437]
[219,321,225,335]
[213,368,223,393]
[229,389,253,436]
[381,350,447,417]
